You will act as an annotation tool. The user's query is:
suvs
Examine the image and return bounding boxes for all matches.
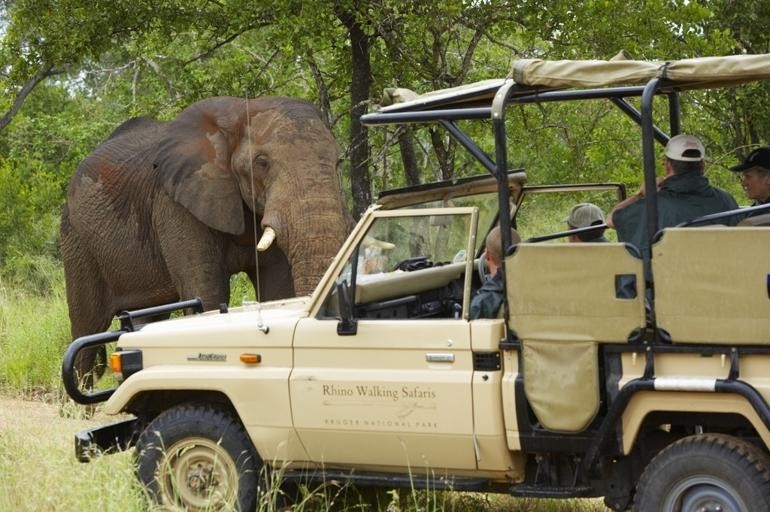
[61,55,770,510]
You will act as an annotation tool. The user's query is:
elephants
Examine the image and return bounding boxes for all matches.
[60,96,395,423]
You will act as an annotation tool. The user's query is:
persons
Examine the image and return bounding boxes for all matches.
[729,146,770,219]
[470,226,523,320]
[558,200,609,242]
[606,134,742,279]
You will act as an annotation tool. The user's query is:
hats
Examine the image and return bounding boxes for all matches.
[560,203,605,228]
[658,135,706,162]
[728,147,770,173]
